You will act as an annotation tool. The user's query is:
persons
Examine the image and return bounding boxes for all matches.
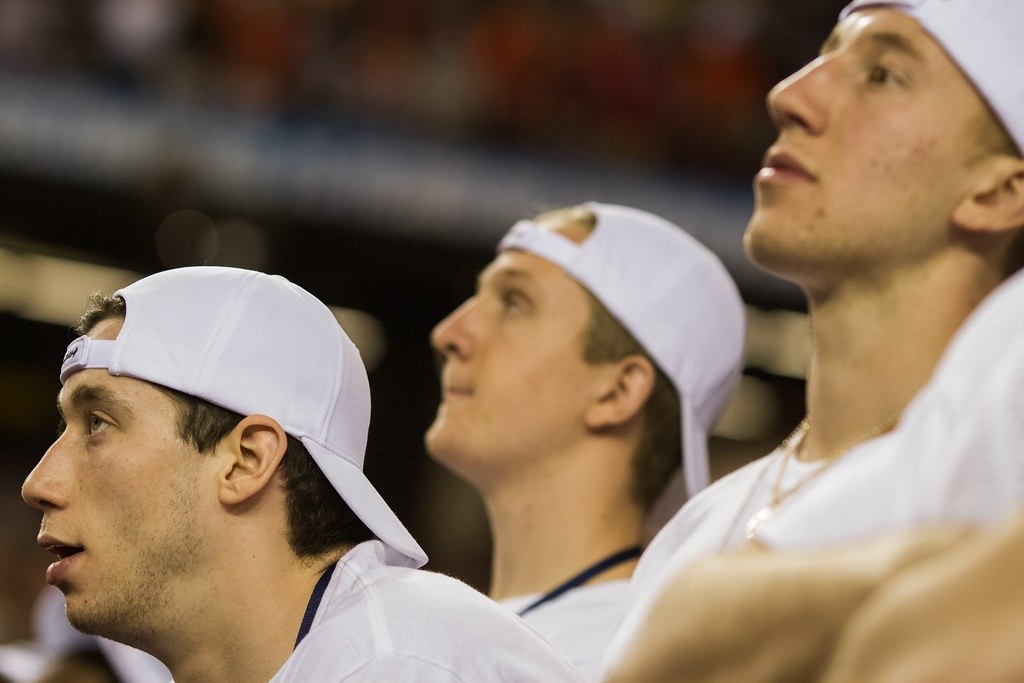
[594,0,1024,682]
[21,266,590,683]
[422,200,747,683]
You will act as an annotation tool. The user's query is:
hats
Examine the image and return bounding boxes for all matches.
[497,201,746,502]
[838,0,1024,156]
[59,265,427,567]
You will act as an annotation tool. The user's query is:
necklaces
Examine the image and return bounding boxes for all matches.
[515,541,645,618]
[716,404,906,557]
